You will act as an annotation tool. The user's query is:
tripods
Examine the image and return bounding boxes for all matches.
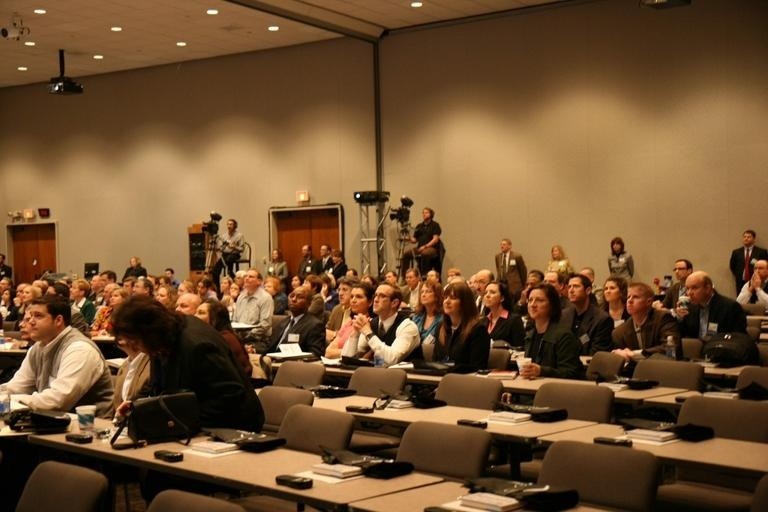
[391,228,421,281]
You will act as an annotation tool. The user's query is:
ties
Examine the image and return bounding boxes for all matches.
[378,321,385,337]
[744,248,750,282]
[289,318,295,331]
[503,254,506,283]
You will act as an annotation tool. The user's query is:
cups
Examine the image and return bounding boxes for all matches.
[516,358,532,375]
[75,405,97,431]
[679,296,690,310]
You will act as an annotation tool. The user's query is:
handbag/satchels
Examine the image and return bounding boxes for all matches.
[616,417,715,442]
[411,357,473,375]
[9,400,72,434]
[110,391,202,450]
[295,384,357,398]
[698,381,766,401]
[460,476,579,511]
[201,427,287,454]
[318,443,414,479]
[491,391,568,422]
[339,355,374,369]
[594,371,659,390]
[372,387,447,410]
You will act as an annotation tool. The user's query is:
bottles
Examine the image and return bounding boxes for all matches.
[652,274,672,295]
[664,336,676,361]
[0,329,6,350]
[0,384,11,421]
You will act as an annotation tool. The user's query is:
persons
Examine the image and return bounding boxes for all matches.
[729,231,767,304]
[1,254,254,379]
[400,208,447,285]
[211,219,246,291]
[106,292,266,505]
[212,239,768,381]
[0,294,116,512]
[70,316,152,512]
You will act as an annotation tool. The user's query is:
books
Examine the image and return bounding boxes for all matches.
[309,461,365,478]
[190,439,241,453]
[702,391,740,401]
[385,397,414,408]
[624,427,680,442]
[457,488,526,510]
[597,380,630,392]
[488,409,533,424]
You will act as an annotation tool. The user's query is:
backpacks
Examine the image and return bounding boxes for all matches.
[701,331,760,367]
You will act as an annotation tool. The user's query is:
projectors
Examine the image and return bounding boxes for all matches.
[47,81,83,95]
[353,190,391,205]
